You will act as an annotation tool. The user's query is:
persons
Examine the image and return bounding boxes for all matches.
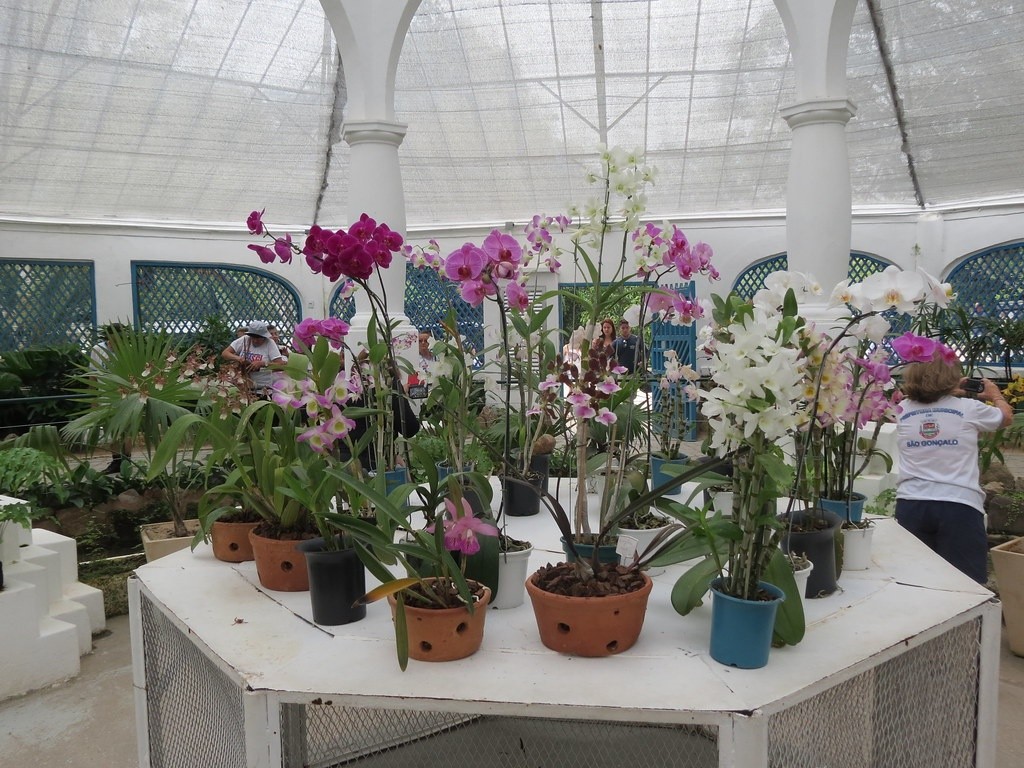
[418,331,439,378]
[892,340,1013,586]
[221,320,293,432]
[592,318,650,412]
[88,323,134,476]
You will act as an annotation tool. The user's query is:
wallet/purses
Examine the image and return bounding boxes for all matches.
[241,361,260,374]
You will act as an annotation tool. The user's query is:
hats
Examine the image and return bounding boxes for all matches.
[244,321,272,339]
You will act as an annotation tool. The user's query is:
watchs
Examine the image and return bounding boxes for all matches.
[265,361,268,368]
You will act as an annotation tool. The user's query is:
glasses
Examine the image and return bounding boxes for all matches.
[623,340,627,348]
[419,339,427,344]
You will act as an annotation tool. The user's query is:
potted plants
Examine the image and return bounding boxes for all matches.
[59,310,205,564]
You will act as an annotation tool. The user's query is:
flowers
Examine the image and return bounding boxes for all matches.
[201,136,987,569]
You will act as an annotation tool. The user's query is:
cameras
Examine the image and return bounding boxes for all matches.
[964,378,984,393]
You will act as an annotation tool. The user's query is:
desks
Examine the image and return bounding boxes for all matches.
[123,502,1002,768]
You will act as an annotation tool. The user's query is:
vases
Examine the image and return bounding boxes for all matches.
[208,493,893,674]
[991,531,1023,658]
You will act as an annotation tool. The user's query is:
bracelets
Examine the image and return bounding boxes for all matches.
[991,394,1004,405]
